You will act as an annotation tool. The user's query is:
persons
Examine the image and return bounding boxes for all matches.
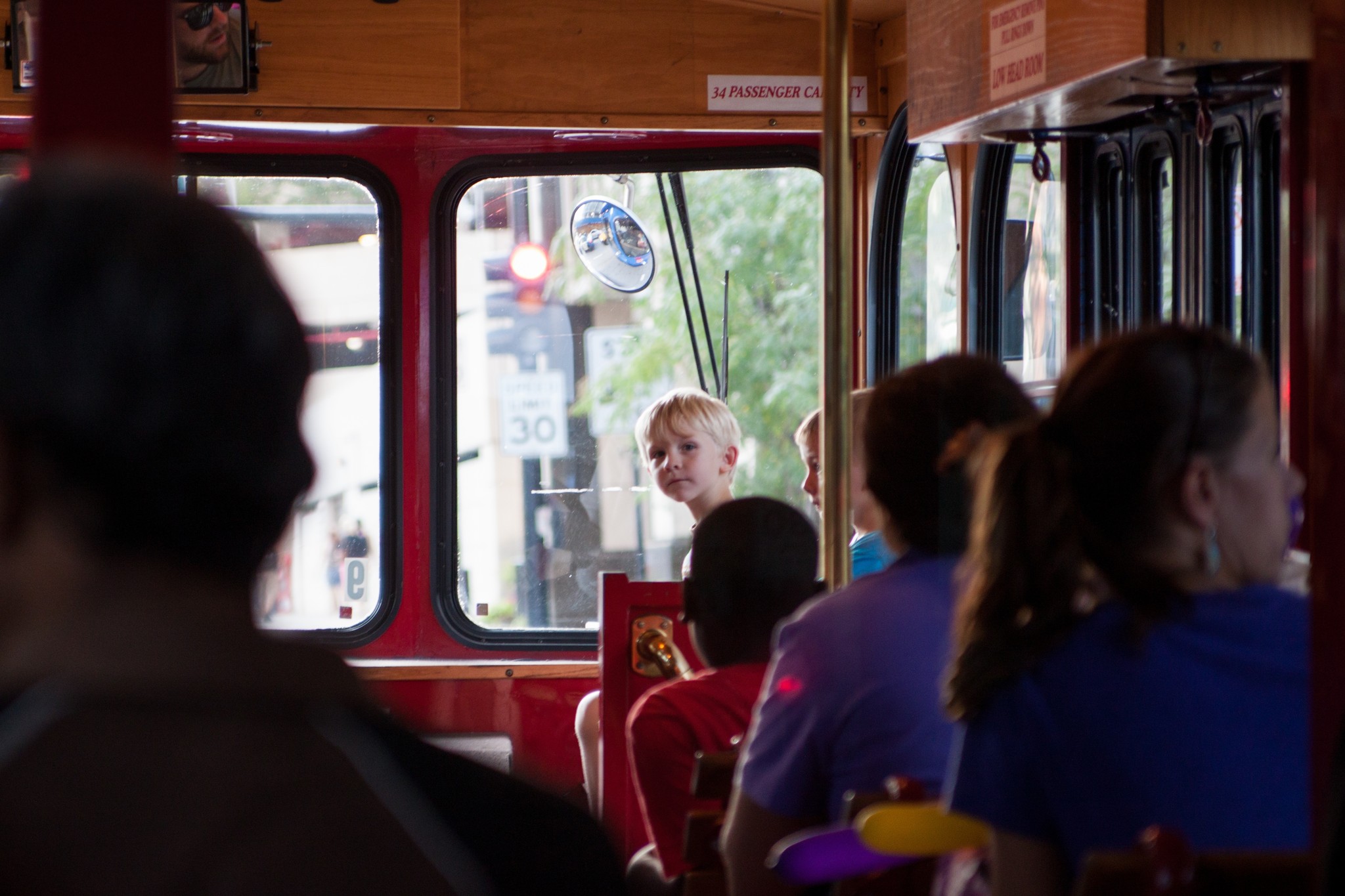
[946,319,1312,896]
[576,389,742,802]
[637,233,646,248]
[625,497,821,896]
[721,357,1046,896]
[174,2,243,87]
[0,178,670,896]
[793,389,895,580]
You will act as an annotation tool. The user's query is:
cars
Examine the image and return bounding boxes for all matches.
[577,225,627,253]
[484,296,575,407]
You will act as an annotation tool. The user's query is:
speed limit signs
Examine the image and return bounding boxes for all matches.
[501,368,569,458]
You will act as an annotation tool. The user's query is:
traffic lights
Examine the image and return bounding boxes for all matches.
[509,240,548,281]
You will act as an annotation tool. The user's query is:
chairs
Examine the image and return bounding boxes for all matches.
[572,691,1004,877]
